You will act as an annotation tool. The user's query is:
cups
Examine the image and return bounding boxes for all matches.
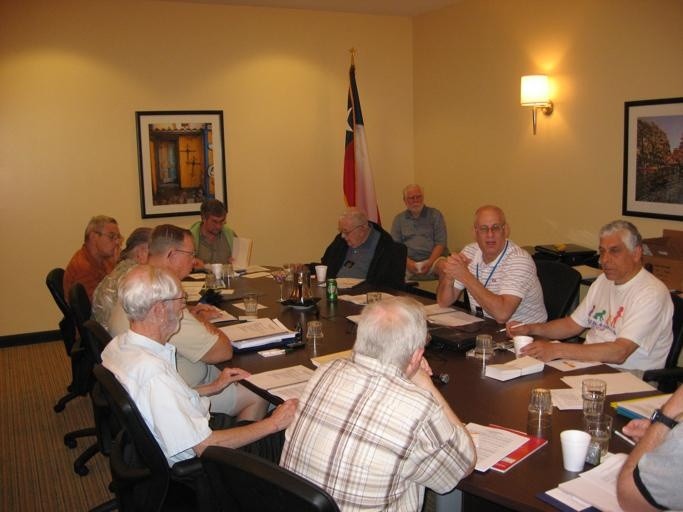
[584,412,613,458]
[560,429,591,472]
[581,379,607,419]
[209,258,381,355]
[584,440,602,472]
[526,385,553,427]
[513,334,533,360]
[474,335,495,358]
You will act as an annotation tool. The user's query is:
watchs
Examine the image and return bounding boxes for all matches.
[648,409,678,428]
[431,372,450,383]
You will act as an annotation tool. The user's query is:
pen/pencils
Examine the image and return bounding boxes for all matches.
[612,428,637,447]
[496,323,527,333]
[563,362,575,367]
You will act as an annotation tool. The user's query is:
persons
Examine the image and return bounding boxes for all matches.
[616,384,683,511]
[61,216,299,481]
[505,219,675,390]
[436,205,549,326]
[190,197,239,271]
[388,184,451,282]
[277,295,477,512]
[319,206,407,291]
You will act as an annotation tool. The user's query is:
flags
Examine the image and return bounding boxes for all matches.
[341,65,383,227]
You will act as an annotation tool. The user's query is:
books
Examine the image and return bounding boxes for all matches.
[310,348,352,368]
[487,423,549,474]
[609,392,675,420]
[463,421,530,473]
[243,364,316,402]
[534,242,599,260]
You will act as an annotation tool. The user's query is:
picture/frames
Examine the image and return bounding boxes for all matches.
[135,111,228,219]
[622,97,683,221]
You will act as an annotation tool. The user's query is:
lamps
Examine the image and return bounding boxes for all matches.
[522,75,552,135]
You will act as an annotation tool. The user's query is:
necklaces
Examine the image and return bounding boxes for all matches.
[351,245,361,255]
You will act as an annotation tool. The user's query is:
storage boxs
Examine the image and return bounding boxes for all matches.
[641,229,683,291]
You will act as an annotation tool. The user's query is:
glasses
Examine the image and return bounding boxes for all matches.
[161,292,188,304]
[93,230,122,242]
[476,224,506,235]
[174,248,197,259]
[338,224,361,235]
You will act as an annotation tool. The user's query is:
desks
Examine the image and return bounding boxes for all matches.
[182,265,666,512]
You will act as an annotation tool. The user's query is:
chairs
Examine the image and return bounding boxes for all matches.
[533,257,583,321]
[395,240,407,289]
[644,293,683,392]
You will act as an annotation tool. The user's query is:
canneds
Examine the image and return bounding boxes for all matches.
[326,279,337,301]
[327,301,337,321]
